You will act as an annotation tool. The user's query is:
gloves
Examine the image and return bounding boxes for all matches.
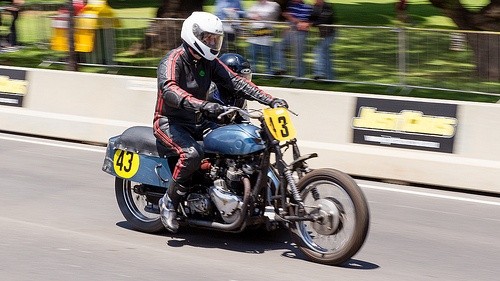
[203,103,225,117]
[270,98,289,109]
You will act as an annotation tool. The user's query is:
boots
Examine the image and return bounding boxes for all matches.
[159,177,187,233]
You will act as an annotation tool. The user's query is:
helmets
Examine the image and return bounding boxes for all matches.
[218,53,252,101]
[181,11,224,62]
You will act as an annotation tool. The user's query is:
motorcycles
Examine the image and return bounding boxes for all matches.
[101,103,370,265]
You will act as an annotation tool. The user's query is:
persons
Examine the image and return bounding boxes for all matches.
[213,0,338,82]
[205,53,252,125]
[152,11,289,233]
